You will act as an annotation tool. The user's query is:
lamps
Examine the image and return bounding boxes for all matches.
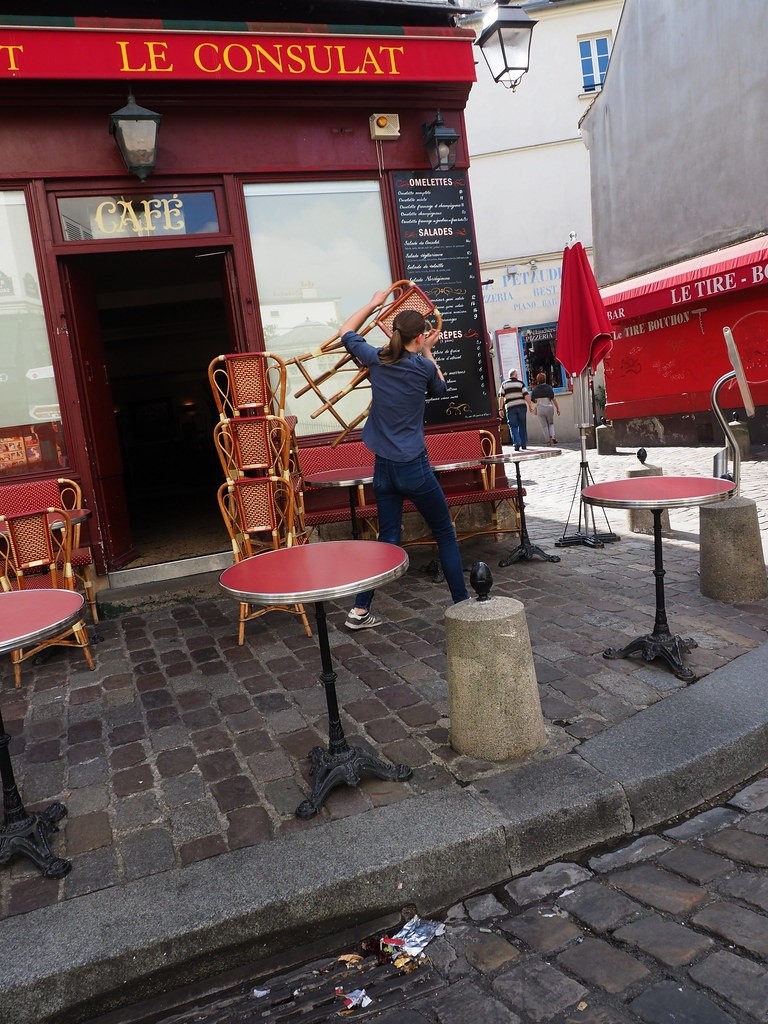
[110,92,160,183]
[422,107,461,172]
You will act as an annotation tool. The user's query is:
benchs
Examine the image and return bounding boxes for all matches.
[294,429,523,545]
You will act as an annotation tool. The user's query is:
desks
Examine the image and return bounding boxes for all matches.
[581,476,738,681]
[0,505,92,579]
[0,588,86,880]
[479,449,562,568]
[409,459,480,583]
[218,540,413,821]
[303,467,376,542]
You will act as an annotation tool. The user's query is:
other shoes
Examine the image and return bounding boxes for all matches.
[522,445,526,449]
[515,447,519,451]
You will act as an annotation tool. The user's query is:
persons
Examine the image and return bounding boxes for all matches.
[531,373,561,447]
[338,290,473,629]
[497,368,534,451]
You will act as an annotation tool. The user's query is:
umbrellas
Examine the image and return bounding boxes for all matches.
[554,231,622,548]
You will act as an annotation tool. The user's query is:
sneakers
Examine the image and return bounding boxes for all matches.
[344,608,383,629]
[547,443,552,447]
[552,438,558,444]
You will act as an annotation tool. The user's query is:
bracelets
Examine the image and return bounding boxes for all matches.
[498,408,503,411]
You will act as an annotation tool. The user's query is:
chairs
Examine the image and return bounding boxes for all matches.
[274,279,443,449]
[0,508,95,689]
[0,477,100,626]
[207,352,316,646]
